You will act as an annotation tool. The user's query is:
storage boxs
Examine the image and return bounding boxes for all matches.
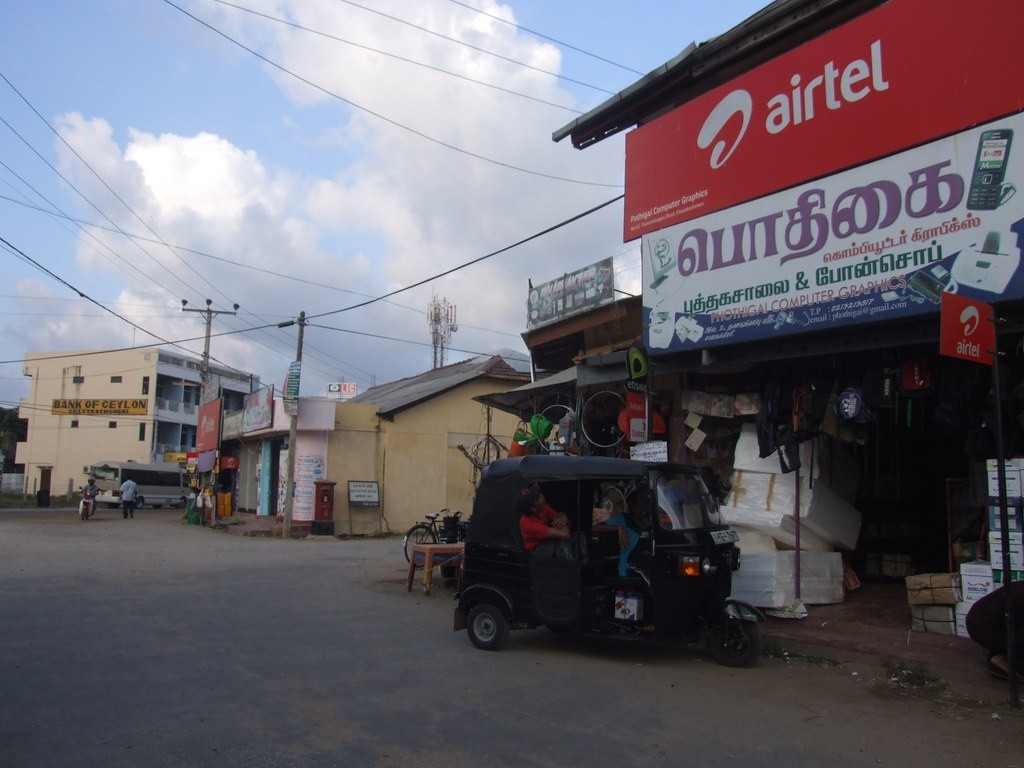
[217,492,232,517]
[720,420,1024,638]
[613,588,643,621]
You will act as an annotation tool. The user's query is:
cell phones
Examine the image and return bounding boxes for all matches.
[967,128,1013,211]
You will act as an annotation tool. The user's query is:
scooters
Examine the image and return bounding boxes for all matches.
[78,485,101,521]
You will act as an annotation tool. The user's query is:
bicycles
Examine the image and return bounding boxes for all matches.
[402,508,465,569]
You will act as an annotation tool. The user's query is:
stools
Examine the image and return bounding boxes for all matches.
[406,544,465,596]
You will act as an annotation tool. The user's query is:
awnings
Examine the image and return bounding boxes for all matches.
[471,366,577,464]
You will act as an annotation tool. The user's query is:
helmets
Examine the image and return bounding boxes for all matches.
[87,478,95,483]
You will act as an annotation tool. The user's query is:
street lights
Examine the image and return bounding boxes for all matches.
[277,310,308,540]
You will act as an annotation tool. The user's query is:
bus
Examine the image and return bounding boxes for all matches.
[89,460,193,509]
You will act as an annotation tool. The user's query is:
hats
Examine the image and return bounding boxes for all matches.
[514,483,540,512]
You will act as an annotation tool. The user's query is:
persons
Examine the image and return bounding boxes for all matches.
[518,482,571,558]
[77,477,98,516]
[591,487,640,576]
[119,474,138,519]
[965,580,1024,683]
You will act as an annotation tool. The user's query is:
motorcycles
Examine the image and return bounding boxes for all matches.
[451,454,769,668]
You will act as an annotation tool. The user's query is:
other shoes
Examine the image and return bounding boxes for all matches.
[131,512,133,518]
[92,511,94,515]
